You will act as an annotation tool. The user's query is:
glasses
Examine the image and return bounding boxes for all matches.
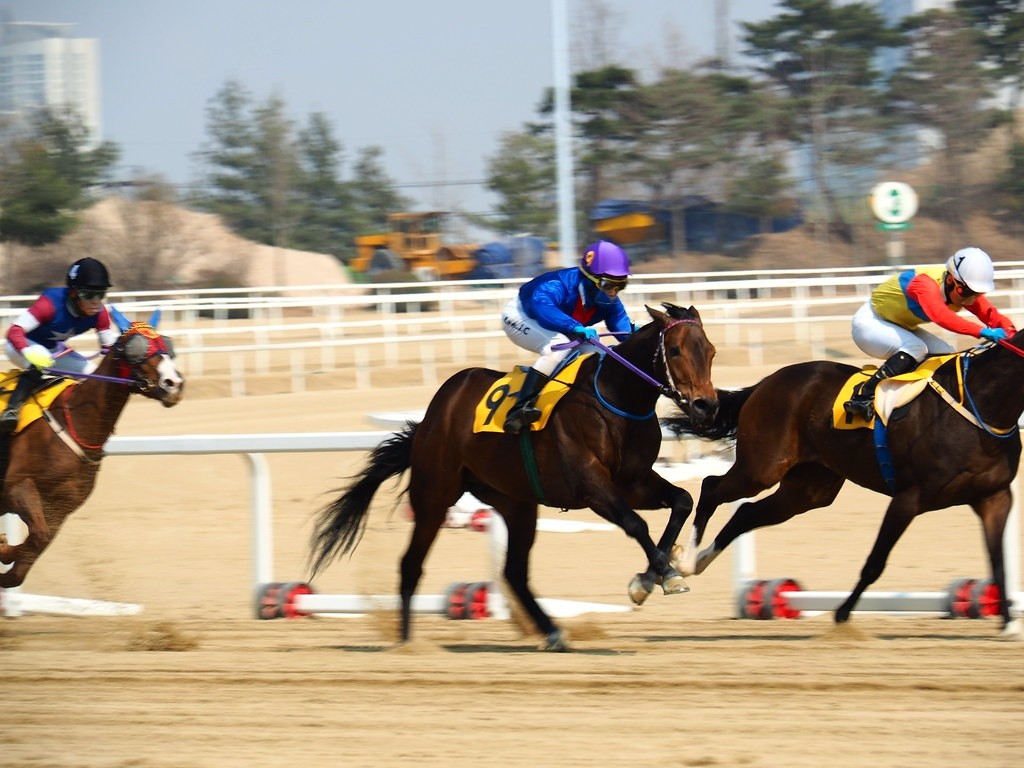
[952,278,980,297]
[588,275,628,291]
[74,288,105,302]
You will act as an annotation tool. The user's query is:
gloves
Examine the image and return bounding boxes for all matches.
[571,324,599,346]
[976,327,1007,350]
[22,344,56,374]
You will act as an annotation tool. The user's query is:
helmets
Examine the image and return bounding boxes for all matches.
[946,247,995,294]
[579,239,630,279]
[66,257,113,290]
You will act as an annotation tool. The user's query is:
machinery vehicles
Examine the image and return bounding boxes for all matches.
[347,210,548,293]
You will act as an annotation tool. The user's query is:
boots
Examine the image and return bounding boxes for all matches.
[503,367,550,433]
[0,364,44,431]
[843,351,918,423]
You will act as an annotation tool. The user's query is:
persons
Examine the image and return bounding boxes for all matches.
[842,246,1019,421]
[503,241,640,436]
[0,257,125,432]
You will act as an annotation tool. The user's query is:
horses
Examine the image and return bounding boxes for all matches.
[0,306,186,588]
[304,302,719,653]
[659,329,1024,636]
